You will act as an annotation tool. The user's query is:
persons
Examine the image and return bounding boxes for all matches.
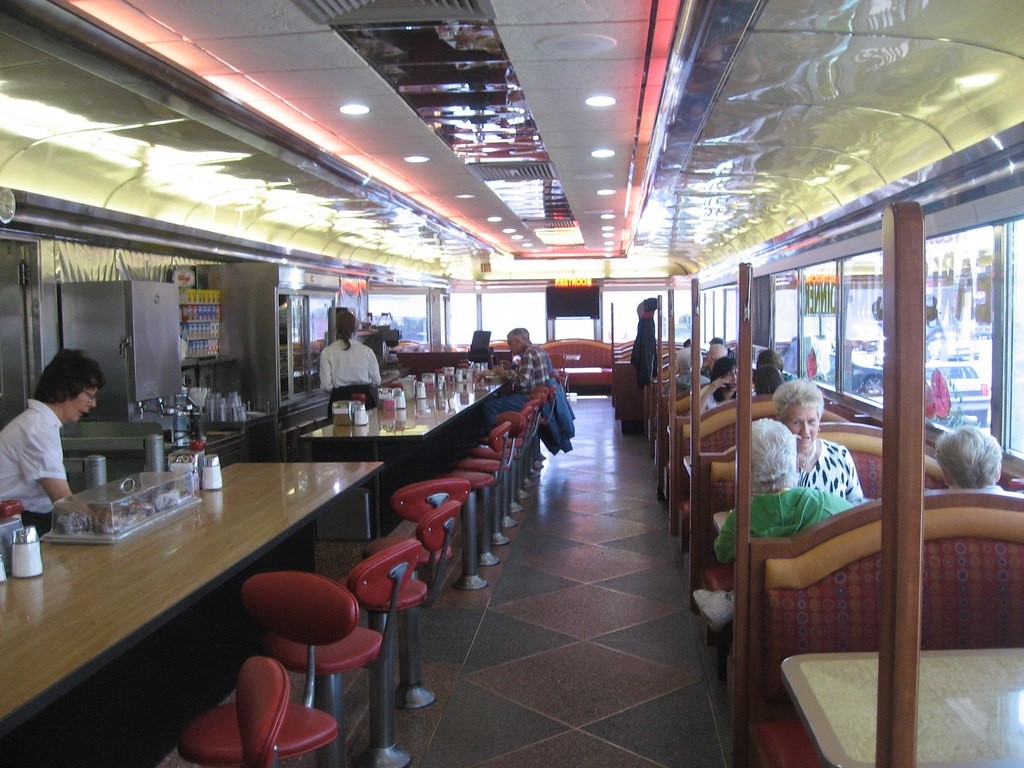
[481,328,553,429]
[0,348,106,538]
[319,311,383,425]
[661,338,1005,563]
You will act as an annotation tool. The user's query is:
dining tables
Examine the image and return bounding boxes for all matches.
[780,647,1024,768]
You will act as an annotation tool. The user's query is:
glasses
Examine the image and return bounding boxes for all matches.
[82,391,97,401]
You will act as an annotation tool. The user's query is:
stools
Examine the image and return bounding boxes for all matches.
[176,382,557,768]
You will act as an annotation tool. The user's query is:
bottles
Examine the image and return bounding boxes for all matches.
[201,454,223,490]
[438,375,444,389]
[354,404,368,425]
[395,388,406,409]
[11,525,43,577]
[377,389,394,410]
[416,382,426,399]
[181,287,221,358]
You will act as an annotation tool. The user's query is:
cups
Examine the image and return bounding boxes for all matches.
[206,391,246,422]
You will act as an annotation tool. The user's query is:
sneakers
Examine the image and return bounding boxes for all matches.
[692,588,735,631]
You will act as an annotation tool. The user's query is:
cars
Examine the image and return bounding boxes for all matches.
[947,337,980,363]
[925,363,992,428]
[831,329,880,352]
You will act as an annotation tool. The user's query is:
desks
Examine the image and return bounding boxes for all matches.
[1,460,388,767]
[301,384,504,540]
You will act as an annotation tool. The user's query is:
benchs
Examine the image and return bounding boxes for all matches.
[538,340,631,386]
[651,364,1023,765]
[489,341,509,350]
